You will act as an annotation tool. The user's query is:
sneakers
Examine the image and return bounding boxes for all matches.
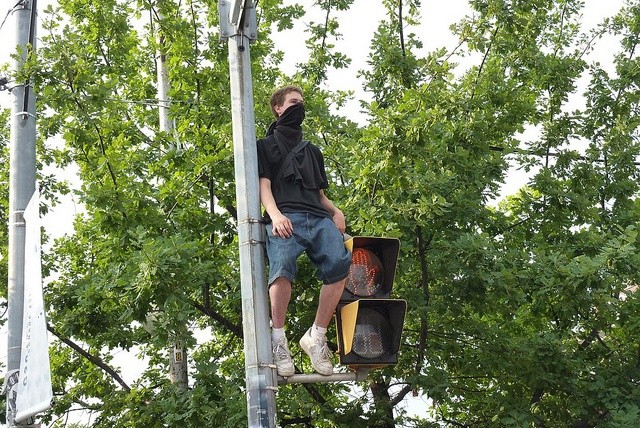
[271,335,295,378]
[300,327,334,375]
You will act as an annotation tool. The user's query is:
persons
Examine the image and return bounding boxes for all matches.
[257,87,351,377]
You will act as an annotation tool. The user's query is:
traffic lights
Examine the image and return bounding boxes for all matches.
[337,235,401,304]
[341,299,408,364]
